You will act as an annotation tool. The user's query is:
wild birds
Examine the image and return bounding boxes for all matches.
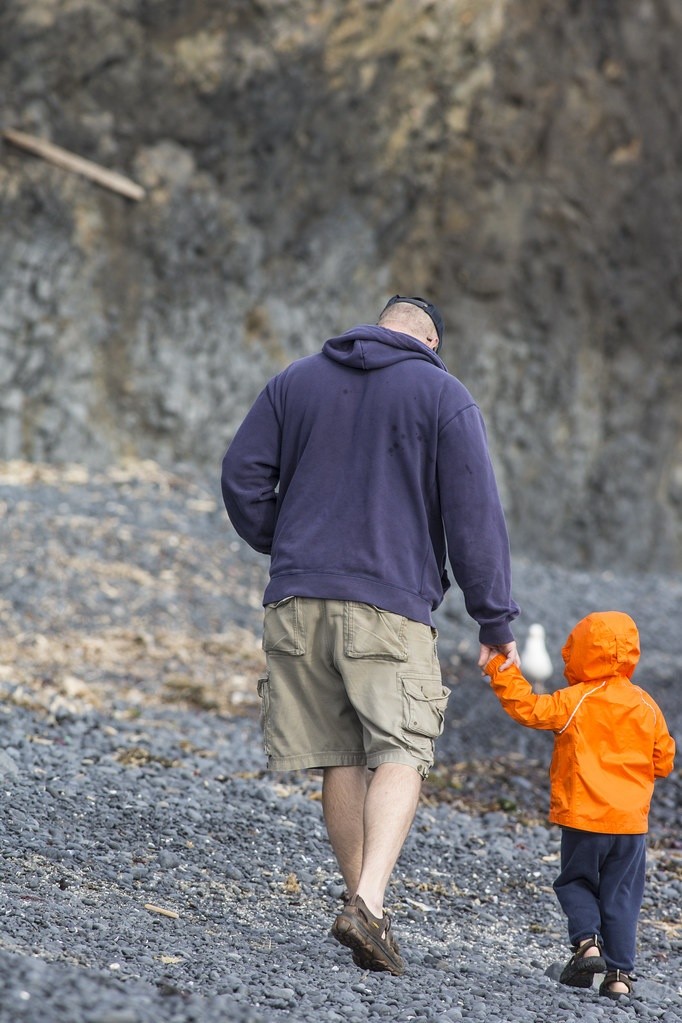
[519,623,554,695]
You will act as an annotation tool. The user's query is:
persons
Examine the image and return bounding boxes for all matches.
[219,294,522,974]
[484,610,677,1002]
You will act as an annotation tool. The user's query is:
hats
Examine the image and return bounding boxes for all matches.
[381,294,443,356]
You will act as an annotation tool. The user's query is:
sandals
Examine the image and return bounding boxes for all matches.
[559,939,606,988]
[332,894,402,976]
[599,971,632,1000]
